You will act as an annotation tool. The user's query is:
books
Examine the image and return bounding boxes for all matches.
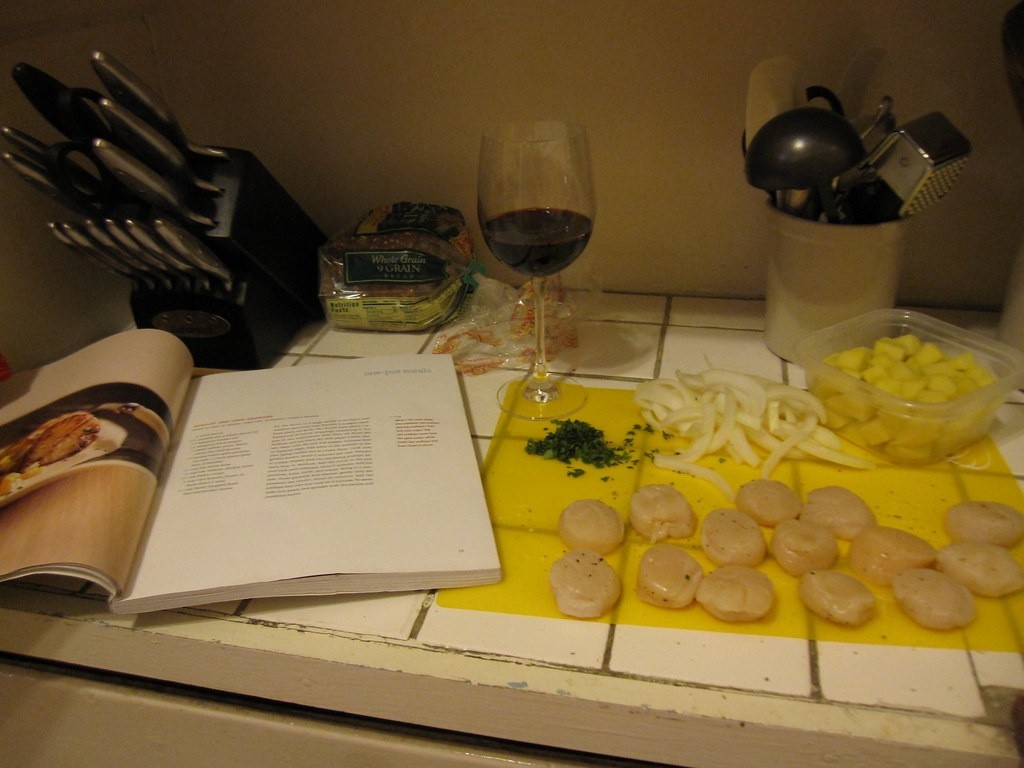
[1,329,502,615]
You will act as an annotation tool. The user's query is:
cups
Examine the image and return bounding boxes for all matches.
[764,200,911,368]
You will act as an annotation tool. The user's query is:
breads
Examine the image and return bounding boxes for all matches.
[324,201,473,333]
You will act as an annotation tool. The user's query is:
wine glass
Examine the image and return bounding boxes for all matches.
[476,122,596,420]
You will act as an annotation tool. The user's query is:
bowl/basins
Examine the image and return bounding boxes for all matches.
[797,311,1023,468]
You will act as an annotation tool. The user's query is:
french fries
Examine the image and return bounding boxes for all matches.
[637,352,877,500]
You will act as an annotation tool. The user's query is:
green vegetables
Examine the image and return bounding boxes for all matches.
[524,418,680,485]
